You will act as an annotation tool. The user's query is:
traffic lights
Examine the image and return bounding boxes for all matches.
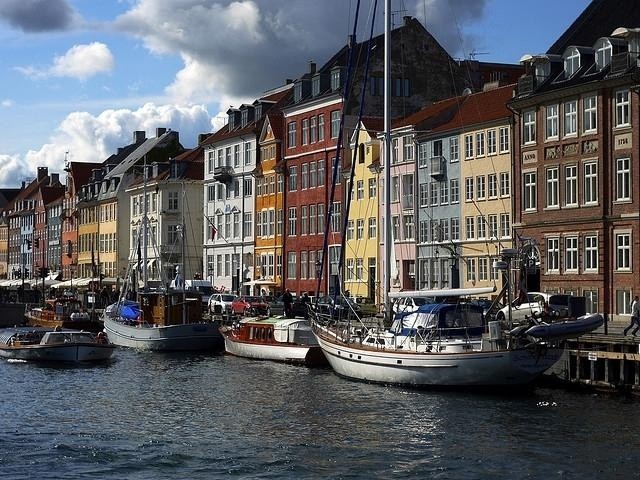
[45,268,50,277]
[28,240,33,250]
[38,268,44,278]
[34,238,40,249]
[25,268,29,279]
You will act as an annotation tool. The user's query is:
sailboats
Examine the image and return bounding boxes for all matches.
[99,148,223,352]
[300,1,605,394]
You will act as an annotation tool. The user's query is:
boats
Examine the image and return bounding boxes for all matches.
[215,314,327,369]
[0,328,117,364]
[23,295,102,330]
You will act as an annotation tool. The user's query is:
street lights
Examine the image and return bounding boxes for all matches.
[68,260,76,290]
[208,265,214,285]
[315,258,321,278]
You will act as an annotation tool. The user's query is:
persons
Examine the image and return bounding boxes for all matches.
[301,293,311,319]
[282,288,293,319]
[54,325,62,332]
[94,287,137,309]
[624,296,640,337]
[95,331,110,344]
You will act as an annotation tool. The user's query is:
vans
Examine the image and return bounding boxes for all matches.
[349,296,379,317]
[303,295,320,311]
[169,278,216,308]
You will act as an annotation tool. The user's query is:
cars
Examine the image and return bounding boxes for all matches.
[495,289,571,324]
[230,295,269,319]
[207,293,238,314]
[470,298,505,324]
[315,295,363,322]
[393,296,437,317]
[258,294,277,304]
[265,295,307,321]
